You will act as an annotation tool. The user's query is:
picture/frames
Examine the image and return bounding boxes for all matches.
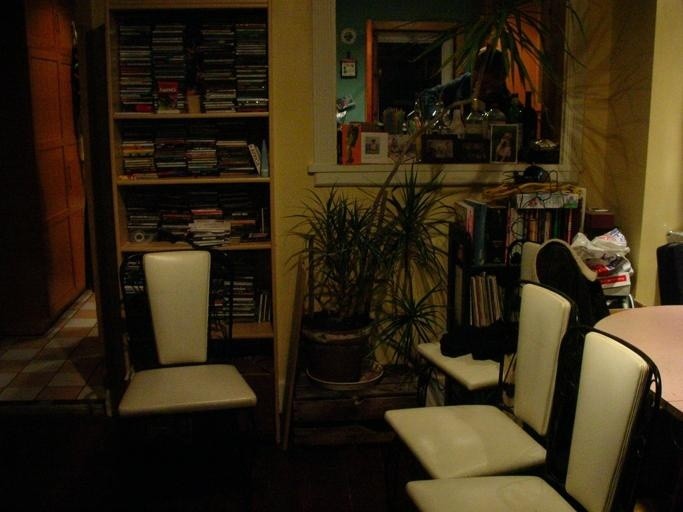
[360,131,389,163]
[421,134,458,164]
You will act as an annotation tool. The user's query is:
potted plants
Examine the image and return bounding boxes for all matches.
[280,154,467,381]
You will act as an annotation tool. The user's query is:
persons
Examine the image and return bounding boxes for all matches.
[416,45,525,124]
[495,131,514,161]
[369,137,378,152]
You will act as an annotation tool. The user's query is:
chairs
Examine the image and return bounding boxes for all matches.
[382,282,575,511]
[404,326,663,512]
[416,237,598,406]
[116,250,259,512]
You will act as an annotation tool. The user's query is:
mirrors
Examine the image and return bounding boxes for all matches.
[307,0,576,172]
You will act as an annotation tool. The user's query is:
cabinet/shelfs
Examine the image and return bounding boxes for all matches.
[101,0,282,445]
[448,220,602,353]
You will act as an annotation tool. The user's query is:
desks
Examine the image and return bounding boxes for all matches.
[591,304,683,512]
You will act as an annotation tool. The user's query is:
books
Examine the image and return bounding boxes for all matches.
[454,200,574,329]
